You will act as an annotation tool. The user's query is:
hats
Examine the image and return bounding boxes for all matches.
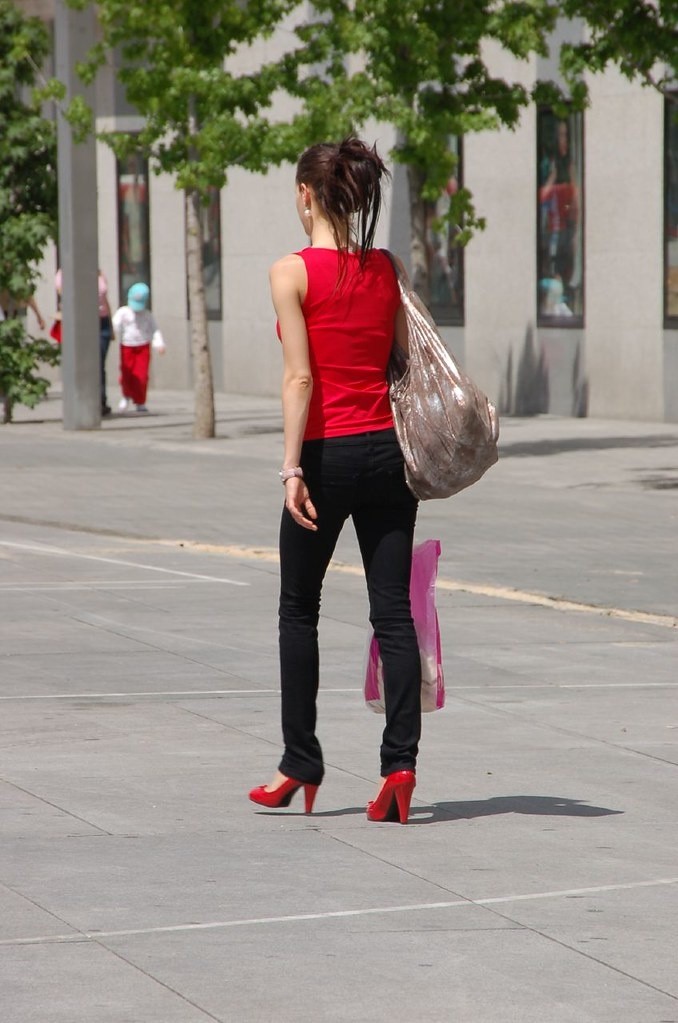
[128,282,151,311]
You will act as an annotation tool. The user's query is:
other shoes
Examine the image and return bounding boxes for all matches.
[118,396,129,408]
[137,404,149,411]
[102,406,111,417]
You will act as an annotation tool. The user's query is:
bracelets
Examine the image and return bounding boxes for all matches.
[278,466,302,485]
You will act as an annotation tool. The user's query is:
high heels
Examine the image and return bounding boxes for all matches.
[249,774,321,814]
[365,770,418,824]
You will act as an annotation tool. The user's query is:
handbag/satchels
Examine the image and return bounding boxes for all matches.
[364,539,445,713]
[373,247,500,501]
[49,320,61,344]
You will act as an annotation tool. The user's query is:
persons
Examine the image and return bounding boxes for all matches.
[121,152,145,282]
[0,288,47,331]
[539,114,577,317]
[111,283,166,411]
[250,140,437,826]
[55,267,115,416]
